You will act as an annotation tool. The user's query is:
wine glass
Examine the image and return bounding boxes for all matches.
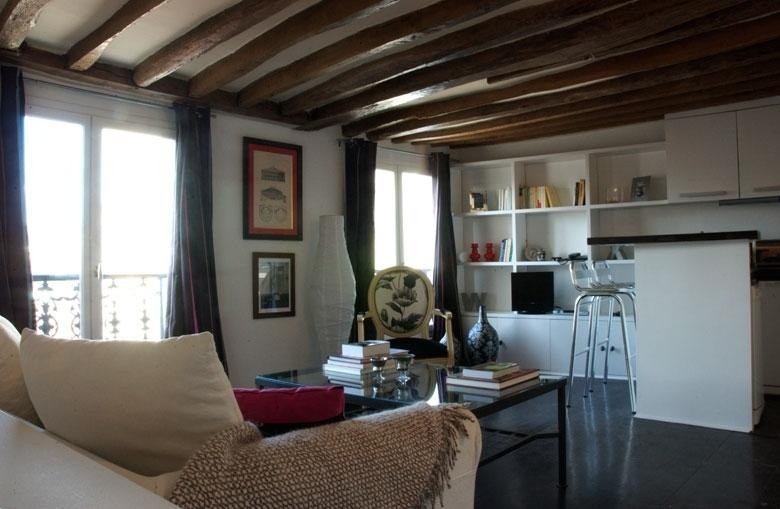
[390,379,413,401]
[369,357,389,381]
[369,383,388,400]
[391,351,416,380]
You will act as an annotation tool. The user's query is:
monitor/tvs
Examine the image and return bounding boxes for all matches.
[511,271,554,314]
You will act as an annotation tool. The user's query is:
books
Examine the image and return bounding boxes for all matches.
[447,378,542,405]
[322,339,414,376]
[446,360,540,390]
[324,369,400,389]
[499,179,587,210]
[498,239,512,262]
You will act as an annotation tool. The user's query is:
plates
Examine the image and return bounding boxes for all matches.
[524,244,542,261]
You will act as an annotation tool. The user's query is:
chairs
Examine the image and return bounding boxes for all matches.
[563,257,638,419]
[586,259,638,395]
[355,264,457,403]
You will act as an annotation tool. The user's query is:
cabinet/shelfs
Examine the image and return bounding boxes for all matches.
[450,137,668,314]
[458,311,552,379]
[659,94,780,207]
[547,315,638,382]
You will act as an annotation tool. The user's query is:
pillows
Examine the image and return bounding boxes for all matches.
[17,324,247,483]
[0,315,47,431]
[230,383,348,437]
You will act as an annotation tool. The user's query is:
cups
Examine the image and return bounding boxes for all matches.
[536,251,545,260]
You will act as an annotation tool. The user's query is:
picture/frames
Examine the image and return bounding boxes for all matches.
[252,252,297,320]
[242,135,304,241]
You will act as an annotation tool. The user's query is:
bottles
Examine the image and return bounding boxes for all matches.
[470,243,481,262]
[484,243,496,261]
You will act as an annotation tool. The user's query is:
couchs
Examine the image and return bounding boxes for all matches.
[0,394,483,508]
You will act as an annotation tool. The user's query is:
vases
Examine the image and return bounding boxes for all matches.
[466,303,500,367]
[308,212,359,373]
[439,331,465,367]
